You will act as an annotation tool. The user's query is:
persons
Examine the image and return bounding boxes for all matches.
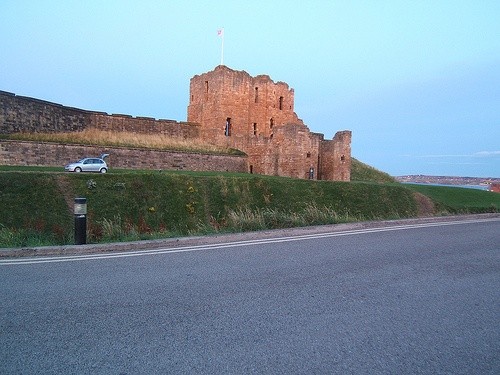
[308,164,313,179]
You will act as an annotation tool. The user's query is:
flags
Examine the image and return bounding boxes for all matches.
[218,28,224,36]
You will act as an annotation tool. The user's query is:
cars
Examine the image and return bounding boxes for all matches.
[64,153,109,173]
